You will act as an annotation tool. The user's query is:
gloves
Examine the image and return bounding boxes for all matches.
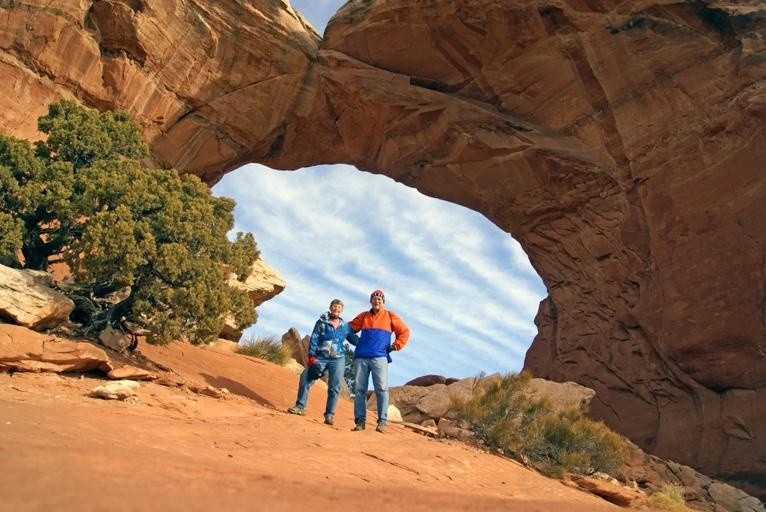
[386,345,395,353]
[306,356,315,366]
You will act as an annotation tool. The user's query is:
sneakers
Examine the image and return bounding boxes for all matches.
[376,422,385,433]
[324,415,334,424]
[354,423,365,431]
[288,406,306,415]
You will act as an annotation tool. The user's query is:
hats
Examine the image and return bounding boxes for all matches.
[308,364,325,380]
[371,289,385,300]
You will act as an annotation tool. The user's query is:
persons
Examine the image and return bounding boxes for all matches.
[286,297,360,426]
[320,288,410,435]
